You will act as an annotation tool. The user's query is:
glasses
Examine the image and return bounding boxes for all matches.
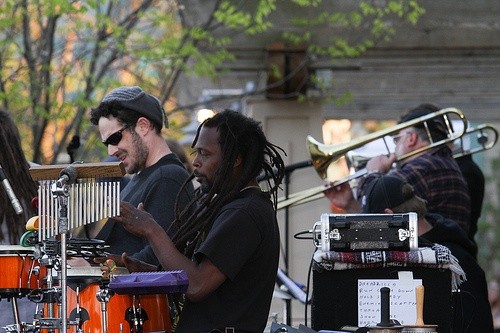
[103,122,138,147]
[393,134,411,145]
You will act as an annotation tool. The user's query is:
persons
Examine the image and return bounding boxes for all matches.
[0,86,288,333]
[322,104,496,333]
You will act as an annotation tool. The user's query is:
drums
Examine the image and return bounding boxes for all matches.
[107,287,172,333]
[41,265,131,333]
[0,245,59,301]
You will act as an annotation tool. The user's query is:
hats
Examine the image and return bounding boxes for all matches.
[101,86,169,132]
[361,172,419,216]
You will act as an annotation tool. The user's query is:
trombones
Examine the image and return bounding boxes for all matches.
[273,107,499,210]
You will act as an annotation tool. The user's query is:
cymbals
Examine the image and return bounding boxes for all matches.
[45,237,111,258]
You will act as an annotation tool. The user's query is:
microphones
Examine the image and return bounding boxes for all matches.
[56,166,78,187]
